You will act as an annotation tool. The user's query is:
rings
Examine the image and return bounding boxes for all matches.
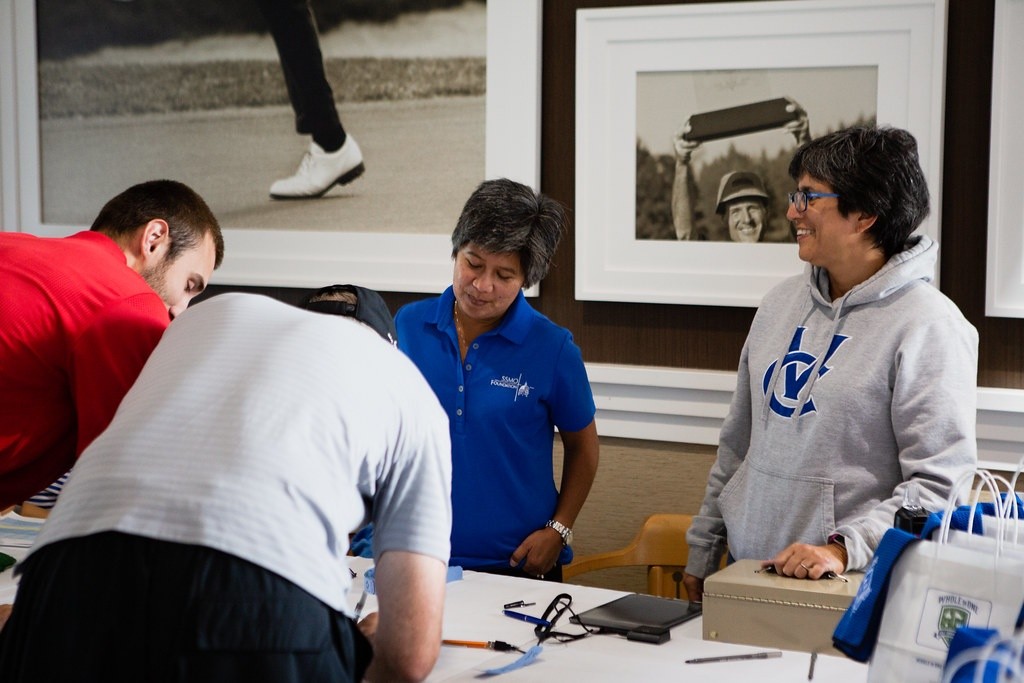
[800,563,809,571]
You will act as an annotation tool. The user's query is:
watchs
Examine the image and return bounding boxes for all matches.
[545,520,573,545]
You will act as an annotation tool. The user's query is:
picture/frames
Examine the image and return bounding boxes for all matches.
[574,0,949,308]
[0,0,541,299]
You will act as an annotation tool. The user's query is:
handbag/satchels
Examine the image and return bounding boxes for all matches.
[867,457,1024,683]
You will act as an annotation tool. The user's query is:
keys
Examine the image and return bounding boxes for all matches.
[754,564,848,582]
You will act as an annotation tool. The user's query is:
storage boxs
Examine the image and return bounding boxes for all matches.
[699,557,866,663]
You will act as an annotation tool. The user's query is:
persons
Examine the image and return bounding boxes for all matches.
[26,468,73,511]
[671,98,813,242]
[391,179,599,582]
[261,0,366,201]
[682,125,979,615]
[0,180,224,512]
[0,284,453,683]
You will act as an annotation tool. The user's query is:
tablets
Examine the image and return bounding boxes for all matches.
[569,594,702,634]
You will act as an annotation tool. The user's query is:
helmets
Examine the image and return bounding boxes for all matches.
[714,170,770,215]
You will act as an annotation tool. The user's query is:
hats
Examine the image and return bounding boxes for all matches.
[306,284,396,346]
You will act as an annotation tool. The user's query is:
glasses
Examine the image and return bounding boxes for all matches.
[788,191,841,212]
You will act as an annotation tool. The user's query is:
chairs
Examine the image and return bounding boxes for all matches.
[560,513,728,601]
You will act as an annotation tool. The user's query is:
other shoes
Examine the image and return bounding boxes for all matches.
[269,132,365,198]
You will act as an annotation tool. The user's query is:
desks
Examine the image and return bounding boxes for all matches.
[348,555,861,683]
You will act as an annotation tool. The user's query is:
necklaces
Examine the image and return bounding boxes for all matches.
[455,300,467,350]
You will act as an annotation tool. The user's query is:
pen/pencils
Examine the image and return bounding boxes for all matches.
[807,650,817,680]
[685,652,784,664]
[353,591,367,625]
[502,609,550,626]
[442,639,494,648]
